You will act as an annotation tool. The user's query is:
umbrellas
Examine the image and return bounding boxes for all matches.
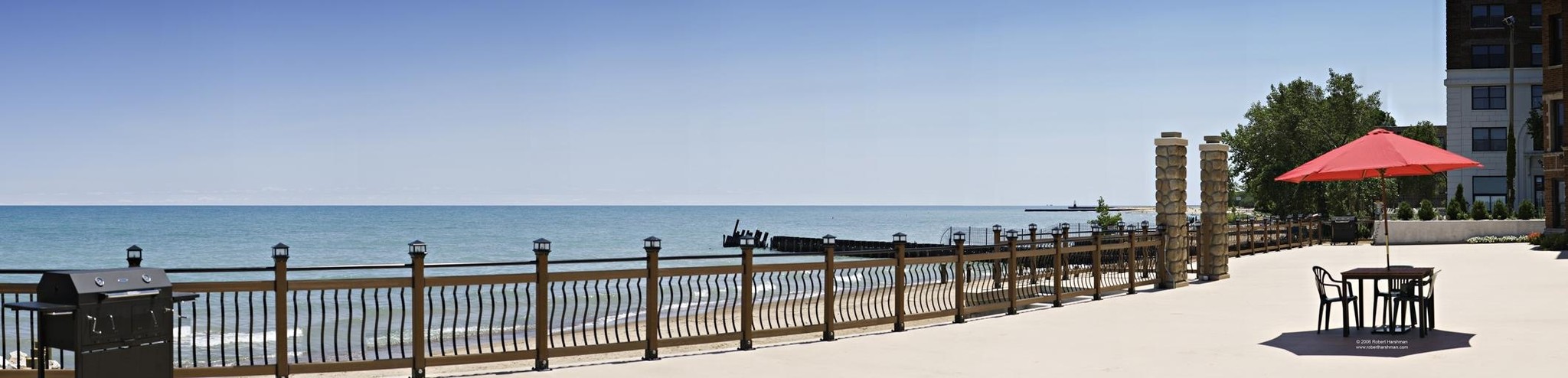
[1273,128,1485,271]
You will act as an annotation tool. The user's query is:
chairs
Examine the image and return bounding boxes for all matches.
[1312,265,1360,334]
[1371,265,1442,335]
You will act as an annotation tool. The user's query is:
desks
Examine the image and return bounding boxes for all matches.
[1339,267,1434,338]
